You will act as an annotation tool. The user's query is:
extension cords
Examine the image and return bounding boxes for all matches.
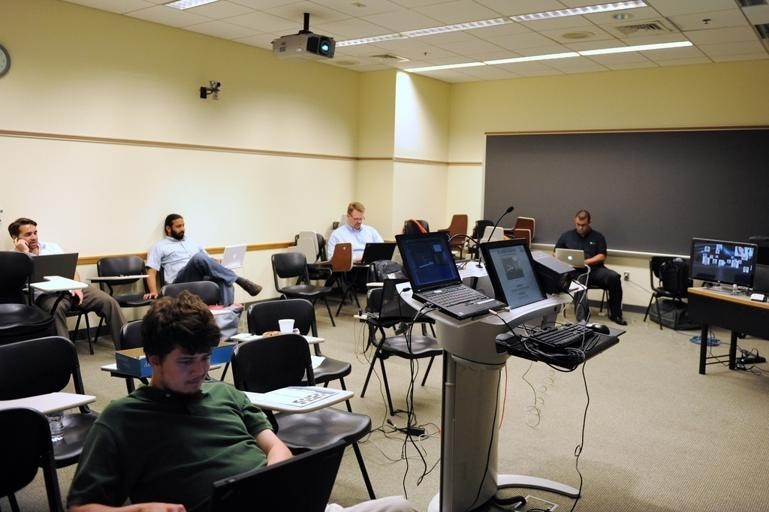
[737,357,765,364]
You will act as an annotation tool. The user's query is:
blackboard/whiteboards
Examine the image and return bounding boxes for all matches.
[483,125,768,260]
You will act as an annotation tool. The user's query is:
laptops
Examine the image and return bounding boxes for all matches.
[30,252,79,283]
[395,231,507,320]
[220,245,247,269]
[210,439,347,512]
[353,243,396,265]
[374,279,435,322]
[555,248,586,268]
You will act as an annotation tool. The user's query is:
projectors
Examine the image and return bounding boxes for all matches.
[272,34,336,60]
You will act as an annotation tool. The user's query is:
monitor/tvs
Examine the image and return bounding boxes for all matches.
[480,239,547,309]
[688,238,758,291]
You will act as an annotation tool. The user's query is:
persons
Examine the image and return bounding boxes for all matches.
[68,289,418,512]
[554,210,628,326]
[9,218,128,350]
[143,213,262,307]
[326,202,385,266]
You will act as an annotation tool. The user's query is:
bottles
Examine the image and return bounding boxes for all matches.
[46,414,63,443]
[733,284,738,294]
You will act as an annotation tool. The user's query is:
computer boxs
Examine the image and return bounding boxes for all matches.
[530,251,576,294]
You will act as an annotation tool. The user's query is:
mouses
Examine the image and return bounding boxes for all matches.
[585,323,609,335]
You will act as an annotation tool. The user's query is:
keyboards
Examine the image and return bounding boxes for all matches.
[529,324,593,349]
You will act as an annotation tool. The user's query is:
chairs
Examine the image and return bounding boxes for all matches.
[229,333,380,504]
[103,316,237,393]
[88,254,157,345]
[141,258,239,307]
[0,250,88,342]
[643,254,691,330]
[248,295,360,415]
[0,333,108,512]
[28,276,99,356]
[267,215,612,416]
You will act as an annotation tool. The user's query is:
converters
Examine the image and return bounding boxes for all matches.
[410,426,425,437]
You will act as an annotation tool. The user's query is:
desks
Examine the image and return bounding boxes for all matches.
[686,285,769,375]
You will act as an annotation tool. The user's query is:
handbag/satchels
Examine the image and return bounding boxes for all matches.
[663,259,692,293]
[651,299,703,330]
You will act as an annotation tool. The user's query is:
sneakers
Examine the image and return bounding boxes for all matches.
[243,280,261,296]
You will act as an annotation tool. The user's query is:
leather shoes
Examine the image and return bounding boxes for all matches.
[610,315,626,325]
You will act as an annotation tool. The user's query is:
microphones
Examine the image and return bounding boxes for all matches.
[476,206,514,268]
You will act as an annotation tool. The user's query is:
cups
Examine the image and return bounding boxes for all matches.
[279,319,295,334]
